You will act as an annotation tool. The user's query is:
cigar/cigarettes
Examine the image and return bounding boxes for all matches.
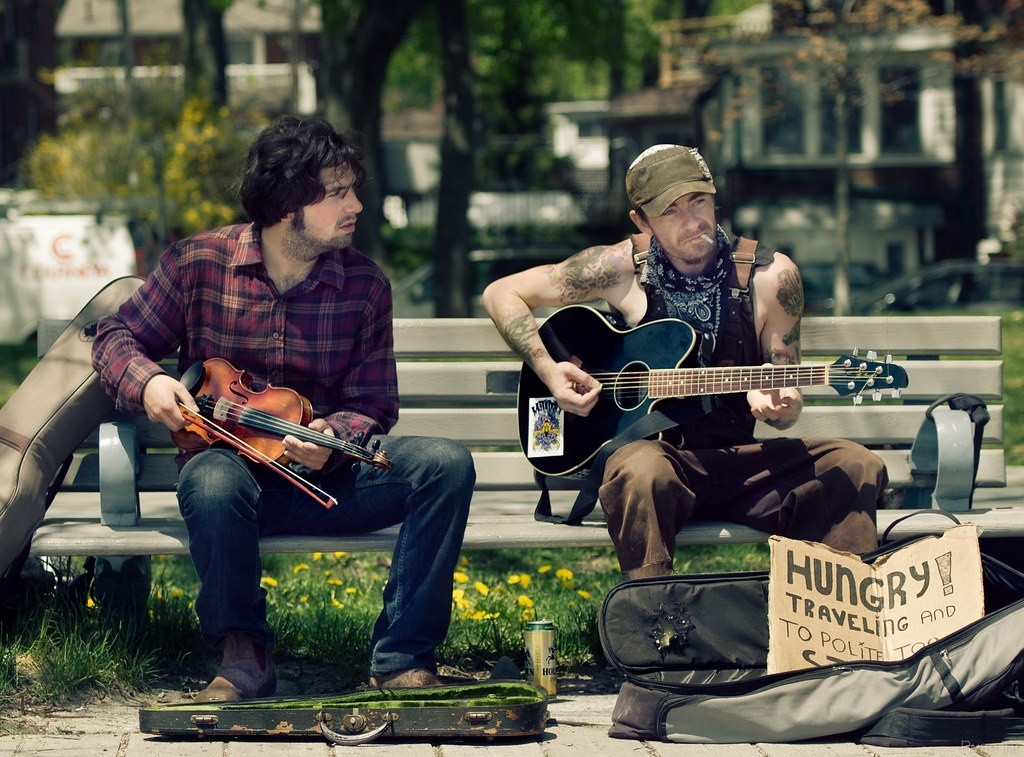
[700,233,716,245]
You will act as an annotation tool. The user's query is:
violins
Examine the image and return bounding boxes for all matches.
[173,356,395,508]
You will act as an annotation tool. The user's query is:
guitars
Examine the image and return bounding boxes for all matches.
[517,303,910,475]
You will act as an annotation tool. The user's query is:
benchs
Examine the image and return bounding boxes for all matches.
[28,318,1024,625]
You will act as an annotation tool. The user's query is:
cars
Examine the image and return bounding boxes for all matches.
[848,255,1024,317]
[384,247,583,302]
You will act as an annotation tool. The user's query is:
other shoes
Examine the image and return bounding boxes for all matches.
[196,641,276,704]
[368,667,444,693]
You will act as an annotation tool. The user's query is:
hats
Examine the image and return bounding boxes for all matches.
[625,144,716,217]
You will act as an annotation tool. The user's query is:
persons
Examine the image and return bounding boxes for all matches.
[92,114,476,704]
[480,144,888,583]
